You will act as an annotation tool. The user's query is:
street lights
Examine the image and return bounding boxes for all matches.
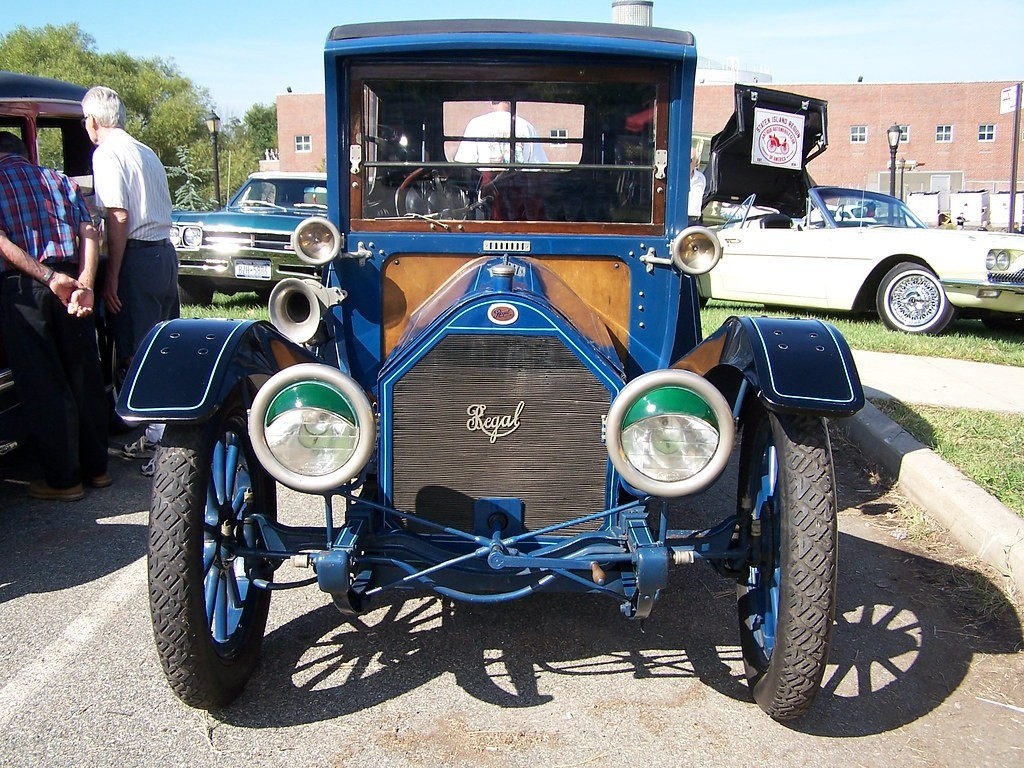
[886,122,903,226]
[899,157,906,200]
[204,109,222,212]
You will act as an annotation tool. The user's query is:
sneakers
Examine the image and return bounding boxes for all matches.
[121,434,154,457]
[141,461,155,476]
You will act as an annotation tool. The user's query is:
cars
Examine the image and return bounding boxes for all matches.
[164,172,330,308]
[694,185,1024,336]
[0,72,126,457]
[114,20,866,721]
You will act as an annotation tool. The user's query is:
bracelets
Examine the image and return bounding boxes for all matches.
[41,268,53,283]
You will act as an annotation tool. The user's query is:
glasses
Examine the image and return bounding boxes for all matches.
[81,117,88,127]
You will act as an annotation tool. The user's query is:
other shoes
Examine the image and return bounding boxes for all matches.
[91,475,111,489]
[28,480,83,501]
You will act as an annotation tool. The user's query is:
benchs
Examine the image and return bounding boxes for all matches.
[394,172,616,221]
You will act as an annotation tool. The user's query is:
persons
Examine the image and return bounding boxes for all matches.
[1012,222,1024,234]
[956,213,966,229]
[864,201,876,217]
[688,147,707,221]
[980,208,990,228]
[938,212,950,225]
[454,100,549,163]
[0,131,111,498]
[82,86,178,459]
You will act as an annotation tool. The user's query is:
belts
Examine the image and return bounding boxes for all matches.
[125,239,170,246]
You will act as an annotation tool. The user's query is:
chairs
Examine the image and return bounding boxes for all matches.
[758,213,794,229]
